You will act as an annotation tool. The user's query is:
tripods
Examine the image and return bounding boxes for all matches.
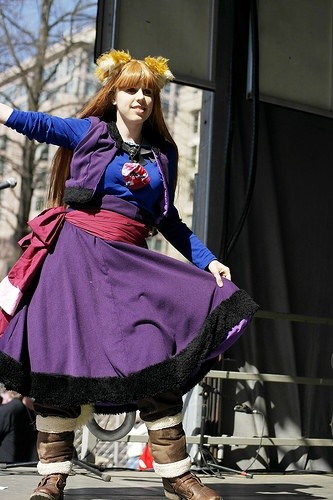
[0,458,111,482]
[191,390,253,479]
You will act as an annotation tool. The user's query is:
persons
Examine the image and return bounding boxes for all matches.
[0,51,259,500]
[0,384,38,468]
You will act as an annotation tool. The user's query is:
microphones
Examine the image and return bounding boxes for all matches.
[233,405,262,415]
[0,178,17,190]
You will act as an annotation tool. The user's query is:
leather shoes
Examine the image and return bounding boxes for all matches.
[162,467,224,500]
[28,473,67,500]
